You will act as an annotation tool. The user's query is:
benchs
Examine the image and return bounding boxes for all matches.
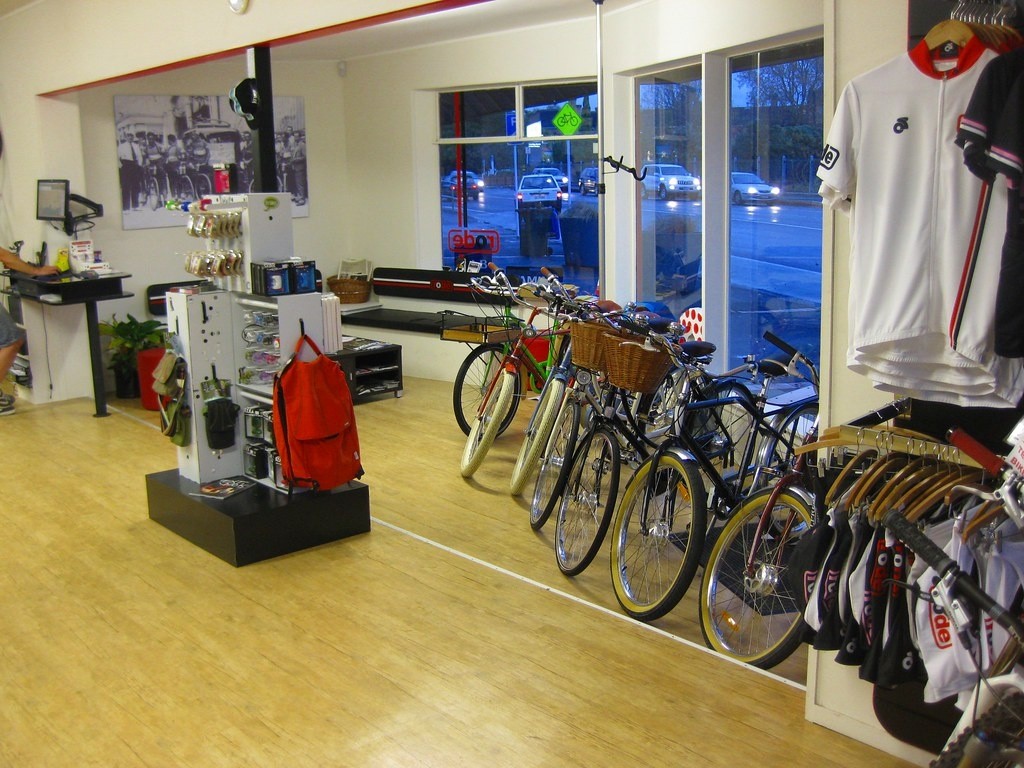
[148,267,322,343]
[341,267,524,386]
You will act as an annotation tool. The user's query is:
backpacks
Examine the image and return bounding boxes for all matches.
[271,335,363,491]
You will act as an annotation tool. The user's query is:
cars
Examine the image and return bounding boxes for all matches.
[532,167,569,192]
[447,171,485,192]
[440,174,480,200]
[578,167,598,195]
[730,172,781,206]
[513,174,562,213]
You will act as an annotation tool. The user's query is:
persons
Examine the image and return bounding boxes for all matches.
[0,246,60,416]
[118,127,310,215]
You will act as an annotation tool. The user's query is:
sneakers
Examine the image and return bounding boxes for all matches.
[0,390,15,417]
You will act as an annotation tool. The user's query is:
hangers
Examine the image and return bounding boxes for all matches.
[793,396,1024,554]
[925,0,1024,52]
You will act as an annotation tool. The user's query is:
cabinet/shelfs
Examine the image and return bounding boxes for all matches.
[326,334,403,406]
[231,291,324,497]
[0,292,89,407]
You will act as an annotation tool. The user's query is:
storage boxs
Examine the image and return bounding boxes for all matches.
[250,258,316,297]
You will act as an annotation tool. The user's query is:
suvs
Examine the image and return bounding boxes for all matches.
[640,165,701,200]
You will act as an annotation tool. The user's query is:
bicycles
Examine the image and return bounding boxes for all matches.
[459,263,687,478]
[138,165,284,212]
[510,276,691,496]
[440,263,563,440]
[554,297,756,578]
[698,324,822,669]
[609,318,820,622]
[881,424,1024,768]
[529,287,720,531]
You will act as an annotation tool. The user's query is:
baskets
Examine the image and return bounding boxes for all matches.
[569,319,640,374]
[325,272,372,303]
[602,328,683,394]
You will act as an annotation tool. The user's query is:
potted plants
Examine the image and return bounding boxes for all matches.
[654,212,702,298]
[558,203,599,269]
[98,312,175,399]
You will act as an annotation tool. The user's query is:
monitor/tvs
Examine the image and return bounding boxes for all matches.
[36,180,103,236]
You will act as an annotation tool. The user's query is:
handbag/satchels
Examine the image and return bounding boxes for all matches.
[152,352,191,448]
